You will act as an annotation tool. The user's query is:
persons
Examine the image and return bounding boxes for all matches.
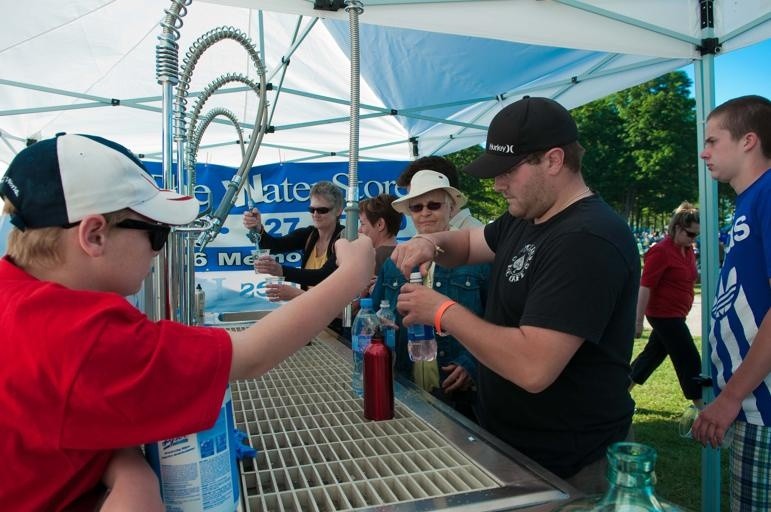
[242,180,346,335]
[396,155,486,230]
[0,129,378,512]
[691,94,771,512]
[266,196,402,302]
[372,170,500,394]
[390,94,642,490]
[630,202,699,404]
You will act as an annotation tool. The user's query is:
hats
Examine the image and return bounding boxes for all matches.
[1,133,202,227]
[466,95,579,179]
[390,169,469,216]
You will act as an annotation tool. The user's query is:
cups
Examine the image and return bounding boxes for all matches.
[251,249,271,274]
[264,276,285,303]
[361,324,397,419]
[352,298,384,396]
[146,384,241,512]
[681,408,735,452]
[376,299,396,389]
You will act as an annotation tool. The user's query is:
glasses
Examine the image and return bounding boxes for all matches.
[408,201,445,212]
[682,228,700,240]
[308,206,333,214]
[111,218,171,251]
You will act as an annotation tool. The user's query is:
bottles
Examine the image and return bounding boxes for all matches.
[592,440,665,512]
[406,270,439,363]
[195,283,205,317]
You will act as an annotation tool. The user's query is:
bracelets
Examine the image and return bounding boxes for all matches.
[411,234,445,260]
[434,299,457,337]
